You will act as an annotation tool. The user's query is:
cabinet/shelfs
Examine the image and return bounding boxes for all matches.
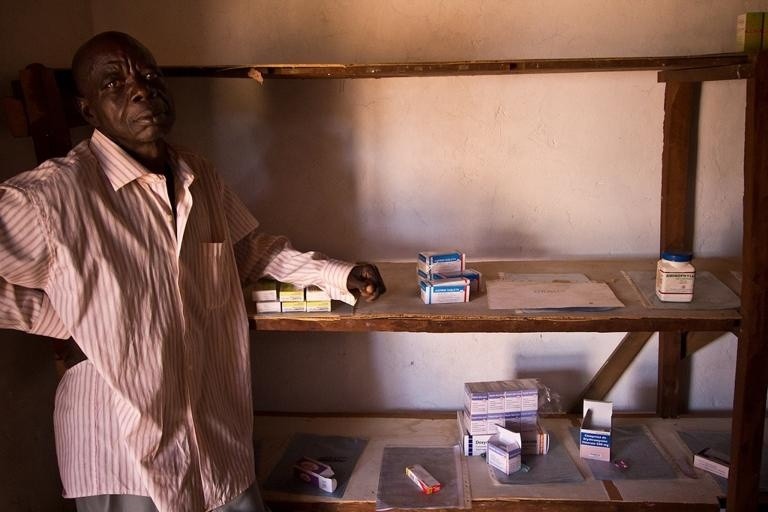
[18,51,767,511]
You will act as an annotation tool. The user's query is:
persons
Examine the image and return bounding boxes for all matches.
[0,31,386,512]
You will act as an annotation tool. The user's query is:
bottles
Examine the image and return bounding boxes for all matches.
[655,248,696,303]
